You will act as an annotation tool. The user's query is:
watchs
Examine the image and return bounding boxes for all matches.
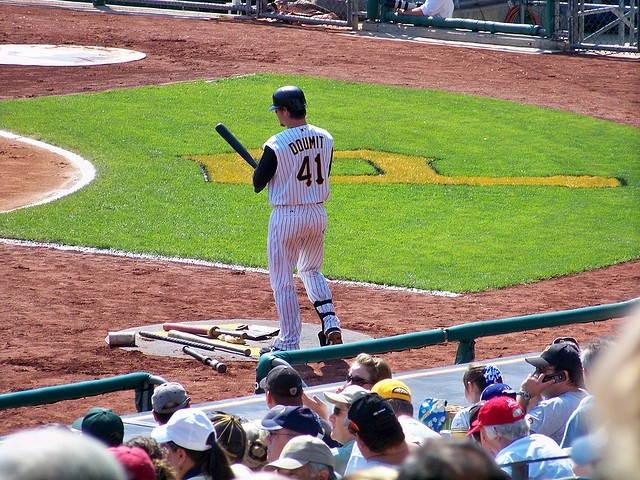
[515,390,531,401]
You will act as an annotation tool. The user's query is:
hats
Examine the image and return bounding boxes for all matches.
[105,445,157,479]
[525,344,583,375]
[151,381,192,414]
[259,364,303,397]
[469,383,516,411]
[150,407,218,452]
[466,395,525,436]
[371,377,413,403]
[209,410,247,465]
[257,405,322,437]
[322,384,370,404]
[264,434,335,472]
[71,408,124,446]
[343,392,395,432]
[418,398,448,434]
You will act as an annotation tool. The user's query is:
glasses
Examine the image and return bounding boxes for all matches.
[333,407,348,415]
[345,374,370,387]
[535,366,554,373]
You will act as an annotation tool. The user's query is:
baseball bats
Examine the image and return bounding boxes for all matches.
[136,320,252,374]
[215,123,258,169]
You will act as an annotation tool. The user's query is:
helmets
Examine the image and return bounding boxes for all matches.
[268,85,308,111]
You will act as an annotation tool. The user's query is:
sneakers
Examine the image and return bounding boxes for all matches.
[323,328,343,346]
[259,346,283,354]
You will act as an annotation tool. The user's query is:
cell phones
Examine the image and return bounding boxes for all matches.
[541,374,565,382]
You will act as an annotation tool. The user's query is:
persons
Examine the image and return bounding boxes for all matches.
[258,363,303,408]
[466,395,575,479]
[127,435,177,479]
[340,392,427,470]
[301,353,391,423]
[203,409,253,480]
[449,364,504,440]
[72,405,158,480]
[260,433,338,480]
[397,436,509,479]
[0,427,130,480]
[256,404,322,463]
[343,377,442,477]
[581,302,640,478]
[253,85,344,356]
[149,381,190,426]
[151,408,237,480]
[514,343,589,442]
[323,385,371,477]
[468,383,517,442]
[240,421,272,469]
[395,0,456,19]
[558,336,623,451]
